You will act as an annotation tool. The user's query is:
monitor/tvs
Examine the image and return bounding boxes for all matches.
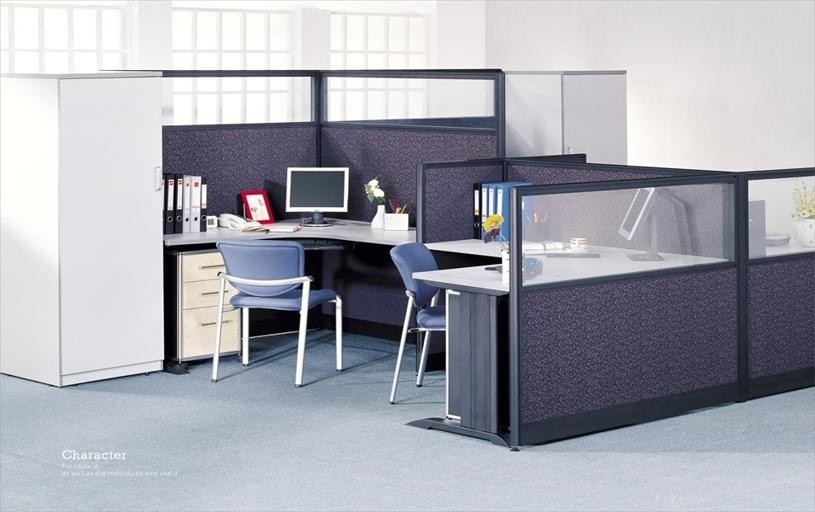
[619,187,663,261]
[285,167,349,227]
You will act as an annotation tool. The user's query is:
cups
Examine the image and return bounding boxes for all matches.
[570,237,587,250]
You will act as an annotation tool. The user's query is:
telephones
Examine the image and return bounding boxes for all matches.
[218,213,248,232]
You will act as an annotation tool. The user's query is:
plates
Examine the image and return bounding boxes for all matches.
[566,246,591,253]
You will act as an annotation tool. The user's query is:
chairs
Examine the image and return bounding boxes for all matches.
[384,244,451,406]
[210,240,345,387]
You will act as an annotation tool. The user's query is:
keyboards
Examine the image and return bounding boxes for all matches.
[296,240,343,247]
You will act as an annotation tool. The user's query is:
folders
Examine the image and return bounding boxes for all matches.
[473,182,533,242]
[162,173,206,234]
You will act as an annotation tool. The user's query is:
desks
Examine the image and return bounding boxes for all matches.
[162,214,731,448]
[747,234,814,404]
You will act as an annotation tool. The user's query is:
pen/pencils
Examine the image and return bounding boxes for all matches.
[526,209,551,224]
[388,198,407,214]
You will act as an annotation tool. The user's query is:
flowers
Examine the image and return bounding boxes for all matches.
[792,182,815,219]
[364,174,388,205]
[482,212,510,248]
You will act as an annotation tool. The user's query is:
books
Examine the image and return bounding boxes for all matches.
[266,223,300,234]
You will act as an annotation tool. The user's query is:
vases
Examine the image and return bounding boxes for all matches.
[370,205,386,229]
[502,248,509,285]
[793,217,815,250]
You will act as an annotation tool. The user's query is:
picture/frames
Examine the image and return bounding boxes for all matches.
[241,188,273,223]
